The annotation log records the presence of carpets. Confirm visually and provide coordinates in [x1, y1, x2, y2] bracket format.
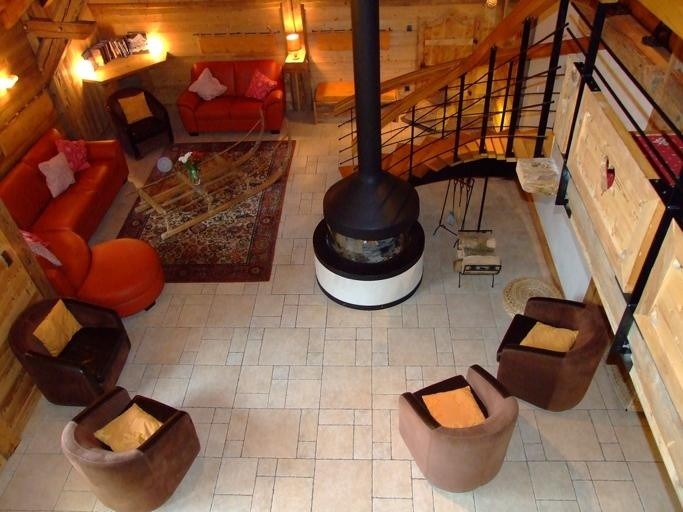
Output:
[503, 277, 565, 319]
[115, 141, 295, 284]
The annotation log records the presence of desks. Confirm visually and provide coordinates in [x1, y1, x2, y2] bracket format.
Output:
[81, 49, 172, 136]
[282, 61, 313, 119]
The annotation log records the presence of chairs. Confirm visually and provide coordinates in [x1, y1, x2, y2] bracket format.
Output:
[8, 297, 132, 407]
[61, 385, 199, 512]
[496, 296, 607, 412]
[452, 230, 500, 287]
[397, 364, 519, 493]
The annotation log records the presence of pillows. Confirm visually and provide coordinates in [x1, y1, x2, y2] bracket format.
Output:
[188, 68, 228, 101]
[243, 70, 278, 99]
[34, 300, 83, 357]
[421, 385, 485, 428]
[107, 87, 175, 161]
[18, 229, 63, 267]
[93, 402, 165, 452]
[520, 321, 579, 353]
[37, 151, 76, 198]
[54, 139, 91, 172]
[118, 92, 155, 125]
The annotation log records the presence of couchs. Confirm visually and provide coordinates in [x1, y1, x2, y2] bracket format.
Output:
[177, 58, 286, 136]
[0, 126, 165, 316]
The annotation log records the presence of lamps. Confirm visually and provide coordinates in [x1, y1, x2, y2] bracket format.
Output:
[285, 34, 302, 60]
